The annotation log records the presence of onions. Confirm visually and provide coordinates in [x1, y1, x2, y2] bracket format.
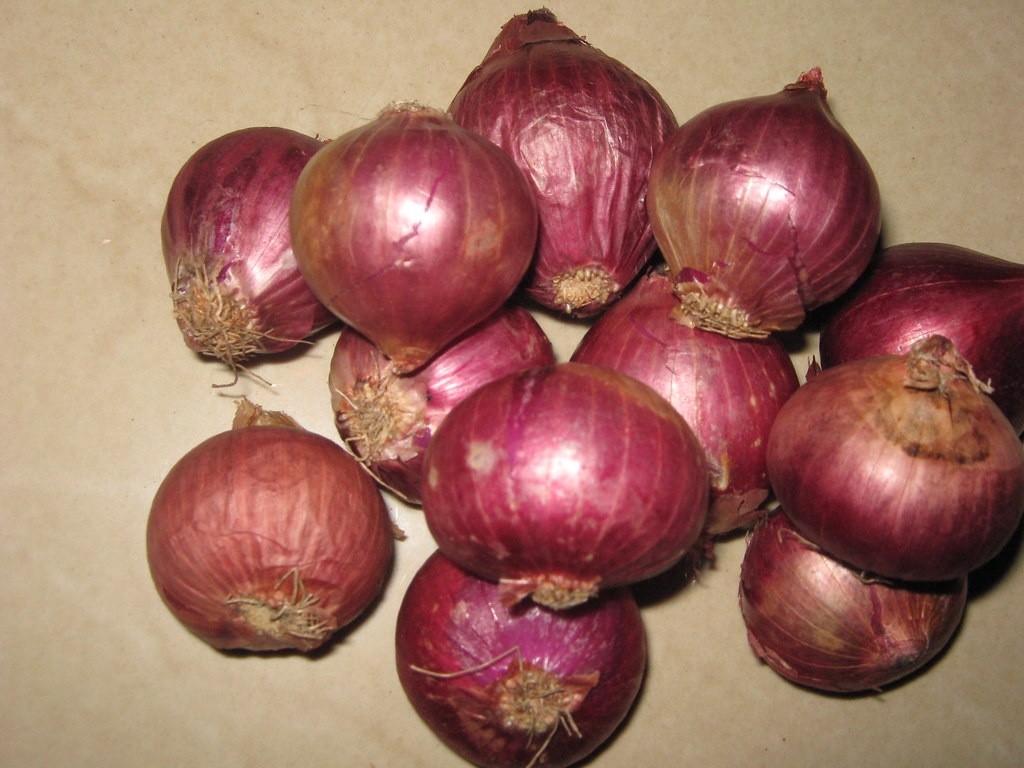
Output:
[145, 7, 1024, 768]
